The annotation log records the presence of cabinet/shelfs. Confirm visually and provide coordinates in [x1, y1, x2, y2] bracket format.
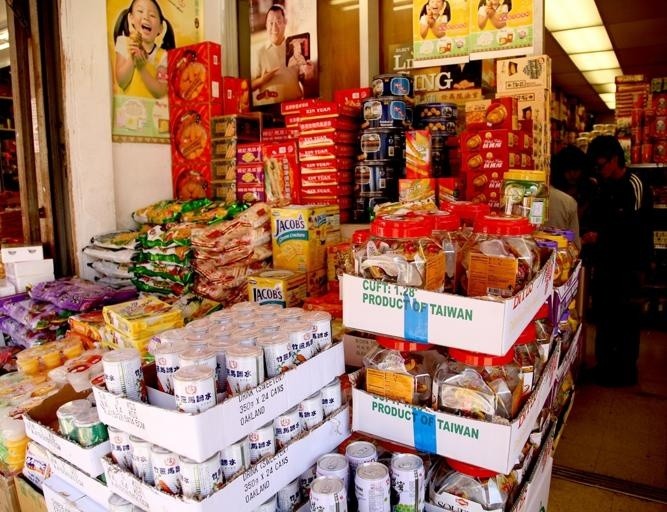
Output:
[625, 163, 666, 329]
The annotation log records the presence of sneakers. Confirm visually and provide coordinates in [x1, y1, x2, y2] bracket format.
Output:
[582, 362, 638, 388]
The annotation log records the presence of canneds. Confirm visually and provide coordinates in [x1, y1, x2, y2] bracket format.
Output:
[55, 301, 426, 512]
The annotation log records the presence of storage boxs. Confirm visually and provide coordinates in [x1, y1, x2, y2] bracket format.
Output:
[168, 43, 554, 220]
[23, 203, 585, 512]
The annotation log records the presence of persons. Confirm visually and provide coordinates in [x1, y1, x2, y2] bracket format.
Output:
[549, 116, 588, 198]
[576, 134, 655, 389]
[557, 142, 600, 327]
[419, 0, 452, 39]
[476, 1, 513, 30]
[255, 3, 308, 79]
[113, 1, 177, 101]
